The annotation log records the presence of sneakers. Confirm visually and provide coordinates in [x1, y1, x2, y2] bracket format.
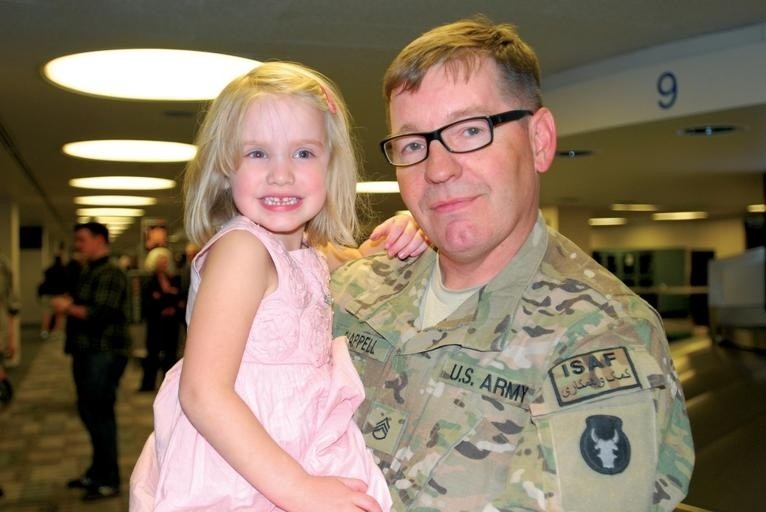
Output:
[66, 471, 120, 504]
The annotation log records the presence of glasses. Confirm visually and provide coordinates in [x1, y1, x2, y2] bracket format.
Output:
[379, 110, 534, 167]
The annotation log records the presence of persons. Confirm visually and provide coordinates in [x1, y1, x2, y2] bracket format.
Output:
[126, 58, 441, 512]
[1, 218, 203, 507]
[324, 19, 697, 512]
